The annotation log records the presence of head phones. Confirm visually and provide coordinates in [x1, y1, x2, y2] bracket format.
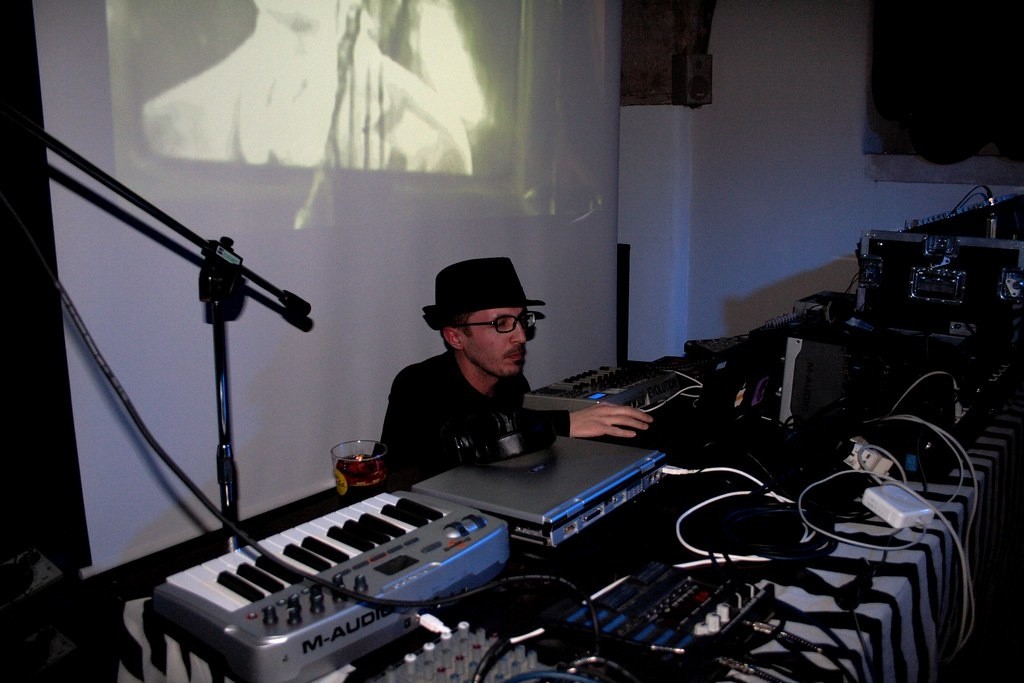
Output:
[439, 409, 558, 465]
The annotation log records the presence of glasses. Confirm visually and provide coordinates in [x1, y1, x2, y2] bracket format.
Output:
[452, 311, 536, 333]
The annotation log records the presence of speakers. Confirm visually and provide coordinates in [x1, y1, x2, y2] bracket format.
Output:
[671, 54, 712, 105]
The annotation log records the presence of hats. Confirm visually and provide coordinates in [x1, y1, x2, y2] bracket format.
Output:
[422, 257, 546, 330]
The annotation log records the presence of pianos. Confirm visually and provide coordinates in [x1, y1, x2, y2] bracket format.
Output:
[150, 490, 509, 683]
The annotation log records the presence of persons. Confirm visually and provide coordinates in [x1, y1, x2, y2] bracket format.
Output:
[380, 257, 652, 481]
[141, 0, 474, 176]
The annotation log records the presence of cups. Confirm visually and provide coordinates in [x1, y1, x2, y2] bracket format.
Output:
[331, 440, 389, 505]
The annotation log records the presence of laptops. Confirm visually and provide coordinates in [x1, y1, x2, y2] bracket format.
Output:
[411, 434, 667, 546]
